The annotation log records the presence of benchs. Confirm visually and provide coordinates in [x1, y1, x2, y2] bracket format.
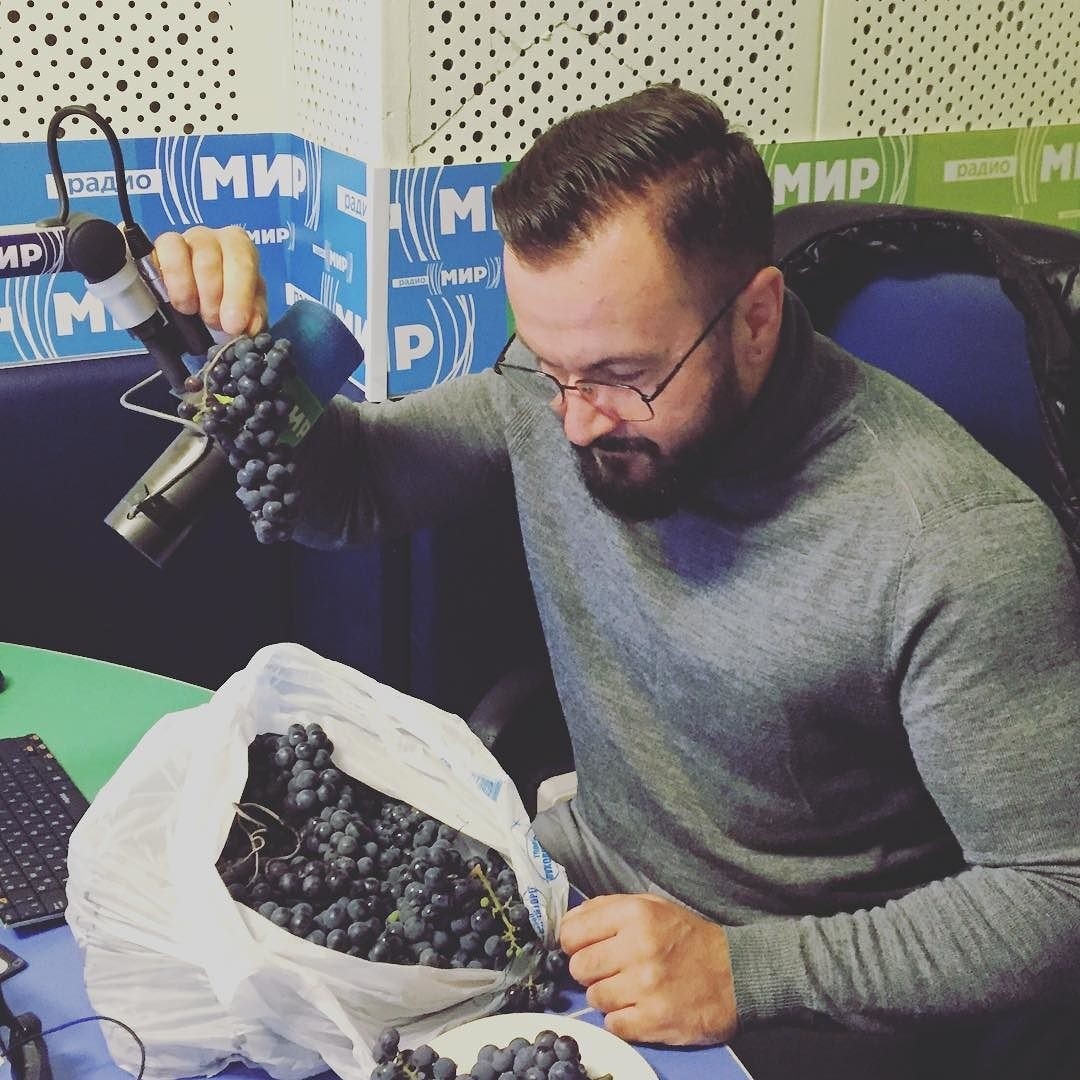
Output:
[1, 640, 757, 1080]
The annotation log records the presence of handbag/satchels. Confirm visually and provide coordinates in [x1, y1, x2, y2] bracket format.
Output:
[64, 644, 568, 1080]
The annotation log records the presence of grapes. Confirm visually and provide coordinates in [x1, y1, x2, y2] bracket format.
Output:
[216, 722, 567, 1015]
[178, 331, 308, 545]
[370, 1026, 613, 1080]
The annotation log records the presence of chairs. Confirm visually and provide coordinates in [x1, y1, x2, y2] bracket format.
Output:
[470, 200, 1080, 807]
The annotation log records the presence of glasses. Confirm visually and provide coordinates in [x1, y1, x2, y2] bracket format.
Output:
[492, 296, 733, 425]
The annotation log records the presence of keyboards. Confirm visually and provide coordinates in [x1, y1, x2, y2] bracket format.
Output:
[0, 733, 92, 925]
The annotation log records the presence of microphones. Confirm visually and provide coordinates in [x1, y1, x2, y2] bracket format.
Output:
[104, 300, 365, 568]
[125, 222, 213, 355]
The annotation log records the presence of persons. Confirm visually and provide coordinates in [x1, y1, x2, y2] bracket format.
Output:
[155, 83, 1080, 1080]
